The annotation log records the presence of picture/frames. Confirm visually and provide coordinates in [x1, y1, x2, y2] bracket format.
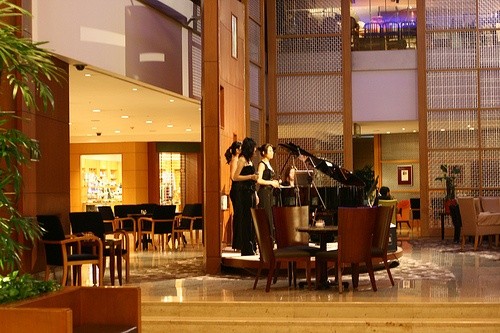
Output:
[396, 164, 413, 186]
[231, 12, 239, 60]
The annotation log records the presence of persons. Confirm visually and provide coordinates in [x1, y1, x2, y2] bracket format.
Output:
[373, 186, 393, 208]
[233, 138, 260, 256]
[280, 165, 298, 186]
[256, 143, 282, 206]
[225, 141, 242, 252]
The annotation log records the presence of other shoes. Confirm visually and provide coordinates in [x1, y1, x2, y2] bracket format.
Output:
[241, 250, 256, 255]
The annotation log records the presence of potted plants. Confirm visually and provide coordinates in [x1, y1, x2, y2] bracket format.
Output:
[434, 165, 462, 214]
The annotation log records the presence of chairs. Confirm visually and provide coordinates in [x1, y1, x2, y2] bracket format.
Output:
[250, 205, 394, 294]
[35, 203, 203, 287]
[457, 197, 500, 251]
[409, 198, 420, 232]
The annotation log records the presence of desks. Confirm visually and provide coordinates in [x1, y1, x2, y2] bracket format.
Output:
[295, 226, 349, 290]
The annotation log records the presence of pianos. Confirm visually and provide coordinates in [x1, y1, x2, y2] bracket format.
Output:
[272, 142, 367, 225]
